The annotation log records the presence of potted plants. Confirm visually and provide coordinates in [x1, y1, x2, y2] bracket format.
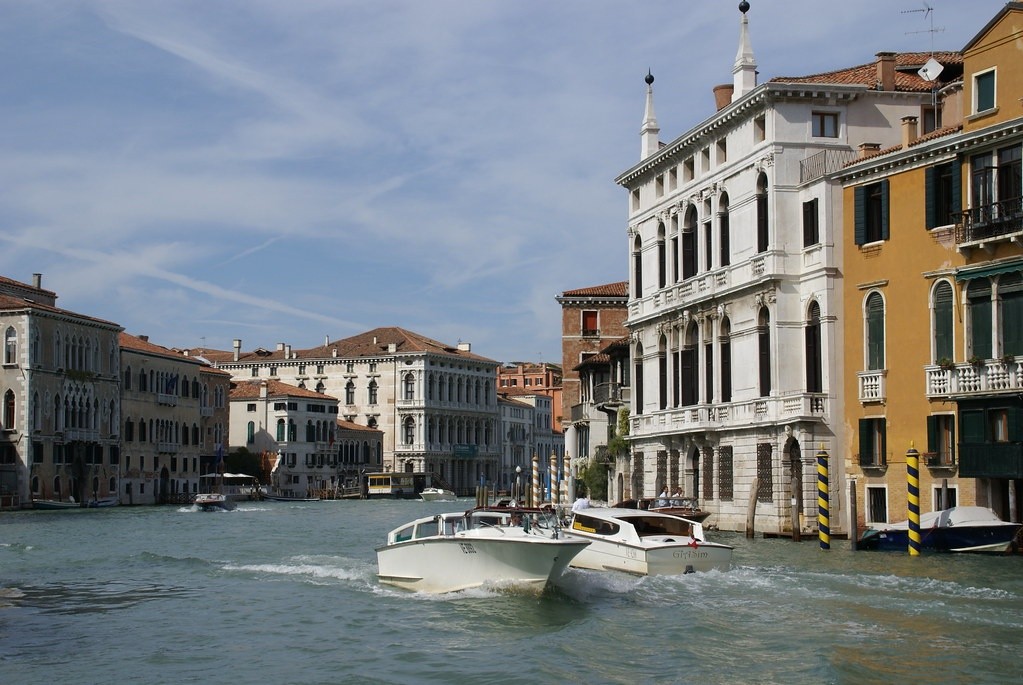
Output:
[935, 357, 954, 371]
[968, 355, 985, 367]
[1000, 354, 1015, 365]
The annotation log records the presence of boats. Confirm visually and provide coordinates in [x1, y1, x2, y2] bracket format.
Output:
[856, 505, 1023, 558]
[32, 497, 118, 511]
[419, 487, 459, 502]
[467, 496, 736, 591]
[373, 500, 592, 600]
[193, 493, 227, 511]
[363, 472, 416, 500]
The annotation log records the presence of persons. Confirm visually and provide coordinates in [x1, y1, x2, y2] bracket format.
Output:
[511, 516, 521, 527]
[659, 485, 670, 508]
[569, 492, 590, 521]
[670, 487, 684, 508]
